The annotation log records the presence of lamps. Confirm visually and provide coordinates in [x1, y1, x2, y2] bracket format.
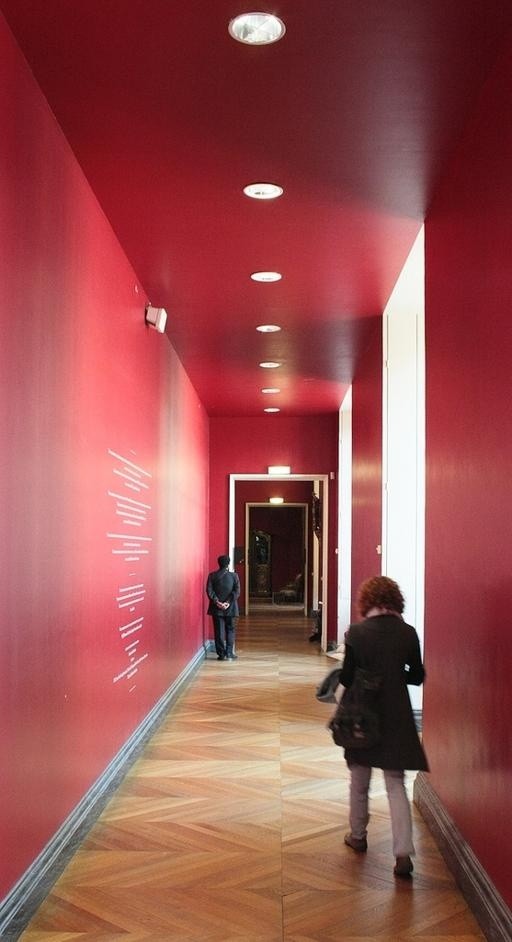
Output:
[144, 305, 168, 334]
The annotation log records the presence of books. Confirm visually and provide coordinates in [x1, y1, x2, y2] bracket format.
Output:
[325, 644, 346, 663]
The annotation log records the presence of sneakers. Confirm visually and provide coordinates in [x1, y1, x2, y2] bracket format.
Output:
[344, 833, 367, 851]
[393, 857, 413, 874]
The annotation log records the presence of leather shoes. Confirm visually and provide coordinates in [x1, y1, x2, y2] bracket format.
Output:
[217, 654, 238, 660]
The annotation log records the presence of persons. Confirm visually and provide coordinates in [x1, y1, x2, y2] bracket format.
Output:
[332, 574, 433, 877]
[204, 555, 242, 661]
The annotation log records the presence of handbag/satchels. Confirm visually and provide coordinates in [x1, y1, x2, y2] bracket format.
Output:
[327, 703, 366, 749]
